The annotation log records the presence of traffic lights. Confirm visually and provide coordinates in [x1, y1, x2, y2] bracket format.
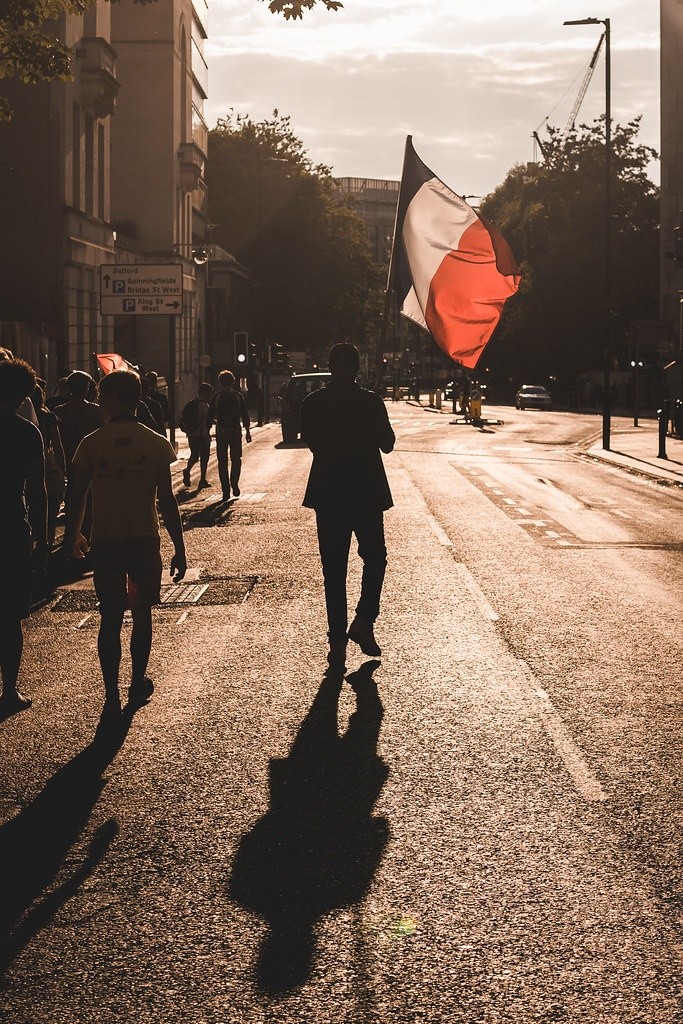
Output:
[234, 332, 248, 366]
[273, 343, 284, 367]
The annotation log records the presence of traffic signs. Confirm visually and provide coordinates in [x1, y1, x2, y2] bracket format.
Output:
[100, 264, 183, 315]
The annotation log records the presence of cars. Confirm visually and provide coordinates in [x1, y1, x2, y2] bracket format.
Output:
[515, 384, 552, 411]
[444, 387, 453, 400]
[280, 373, 337, 444]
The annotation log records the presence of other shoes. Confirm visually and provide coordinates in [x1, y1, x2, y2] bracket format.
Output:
[328, 641, 346, 663]
[101, 701, 121, 720]
[0, 690, 32, 711]
[349, 615, 381, 656]
[223, 491, 230, 500]
[128, 677, 154, 702]
[183, 469, 190, 487]
[199, 482, 211, 488]
[231, 480, 240, 496]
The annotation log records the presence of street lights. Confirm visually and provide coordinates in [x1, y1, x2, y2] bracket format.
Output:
[631, 360, 644, 427]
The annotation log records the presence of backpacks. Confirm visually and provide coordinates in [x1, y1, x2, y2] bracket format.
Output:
[217, 390, 240, 426]
[179, 397, 209, 433]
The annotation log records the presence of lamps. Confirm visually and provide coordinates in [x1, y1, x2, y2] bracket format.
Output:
[174, 242, 207, 264]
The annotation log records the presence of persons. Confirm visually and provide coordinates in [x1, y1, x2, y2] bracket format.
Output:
[455, 369, 466, 414]
[456, 371, 472, 415]
[0, 346, 253, 716]
[298, 343, 395, 667]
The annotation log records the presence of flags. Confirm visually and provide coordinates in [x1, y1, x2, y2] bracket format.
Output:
[95, 352, 140, 377]
[386, 135, 524, 368]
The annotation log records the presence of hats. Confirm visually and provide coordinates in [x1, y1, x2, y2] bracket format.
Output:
[64, 370, 92, 393]
[57, 377, 68, 389]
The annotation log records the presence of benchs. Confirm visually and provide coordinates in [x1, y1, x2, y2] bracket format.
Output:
[385, 386, 410, 399]
